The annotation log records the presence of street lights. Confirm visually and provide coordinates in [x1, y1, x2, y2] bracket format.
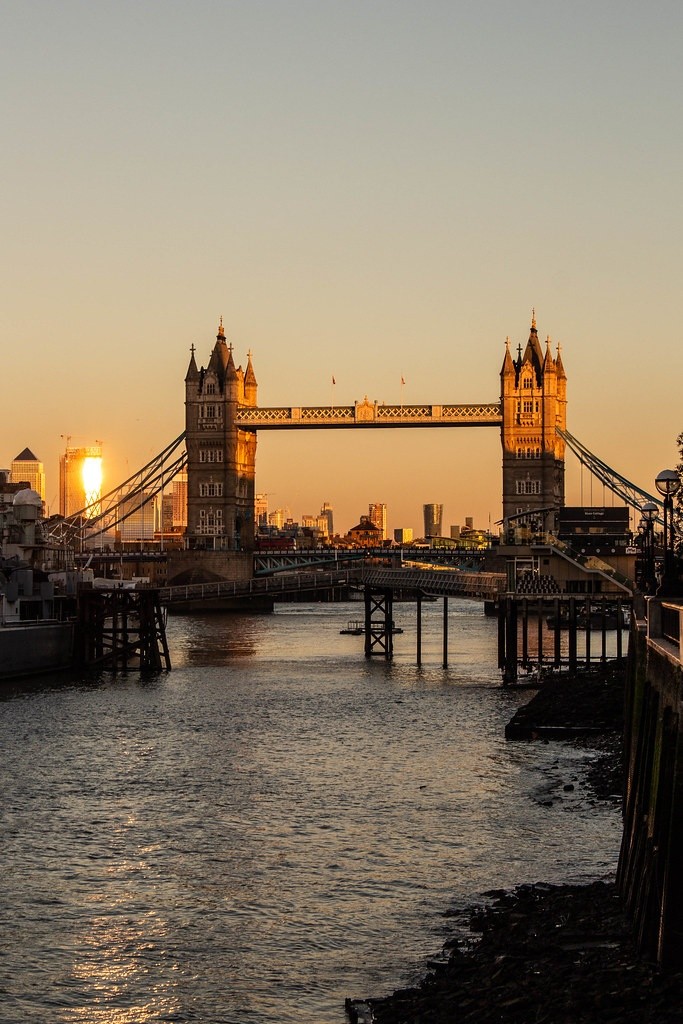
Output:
[639, 517, 649, 578]
[639, 502, 660, 595]
[654, 468, 677, 594]
[636, 525, 645, 581]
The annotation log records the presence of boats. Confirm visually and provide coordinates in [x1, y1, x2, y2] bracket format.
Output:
[546, 604, 638, 630]
[339, 620, 405, 636]
